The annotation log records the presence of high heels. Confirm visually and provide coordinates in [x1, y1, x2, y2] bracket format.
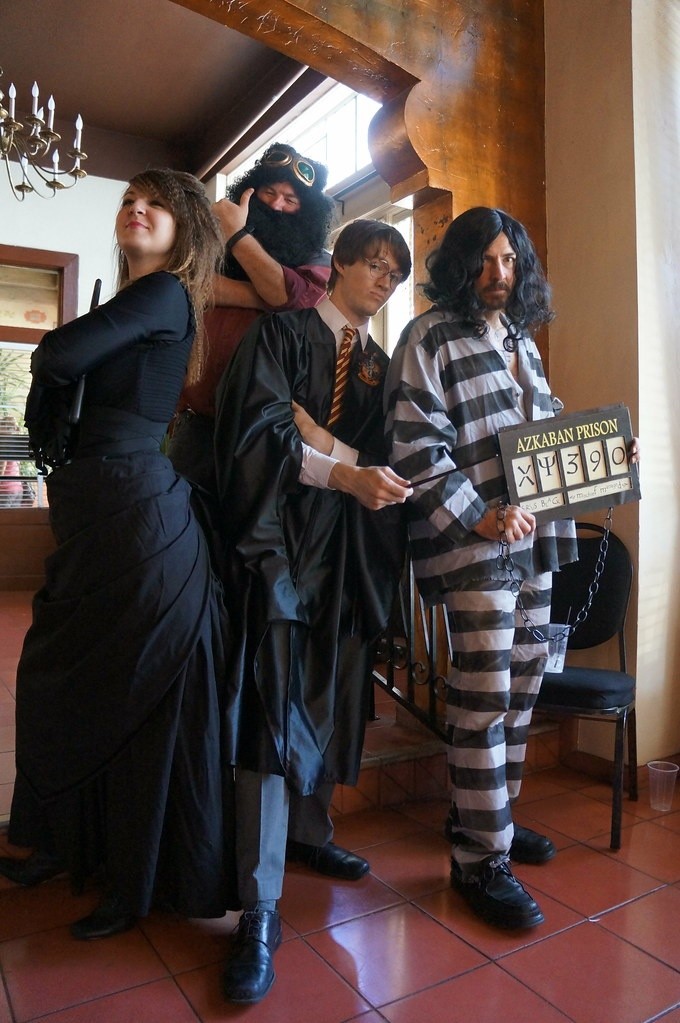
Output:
[64, 884, 164, 943]
[0, 849, 83, 897]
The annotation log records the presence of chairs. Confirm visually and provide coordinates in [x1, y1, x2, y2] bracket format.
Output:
[534, 522, 639, 850]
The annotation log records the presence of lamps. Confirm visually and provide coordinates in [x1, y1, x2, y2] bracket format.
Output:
[0, 66, 88, 202]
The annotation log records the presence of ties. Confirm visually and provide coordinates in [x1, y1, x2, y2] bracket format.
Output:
[323, 324, 358, 432]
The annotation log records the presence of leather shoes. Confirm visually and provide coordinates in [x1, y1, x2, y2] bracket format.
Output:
[218, 908, 283, 1006]
[286, 840, 370, 882]
[449, 855, 545, 932]
[444, 814, 557, 867]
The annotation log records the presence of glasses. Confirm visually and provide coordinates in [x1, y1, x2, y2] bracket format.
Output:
[360, 254, 406, 292]
[263, 150, 317, 187]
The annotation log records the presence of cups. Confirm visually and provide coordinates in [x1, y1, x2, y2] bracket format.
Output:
[647, 760, 679, 811]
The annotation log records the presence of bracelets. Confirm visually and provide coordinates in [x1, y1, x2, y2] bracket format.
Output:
[224, 223, 257, 250]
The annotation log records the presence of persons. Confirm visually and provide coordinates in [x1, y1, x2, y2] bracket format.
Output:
[167, 142, 331, 423]
[384, 204, 640, 936]
[195, 220, 415, 1005]
[1, 169, 229, 942]
